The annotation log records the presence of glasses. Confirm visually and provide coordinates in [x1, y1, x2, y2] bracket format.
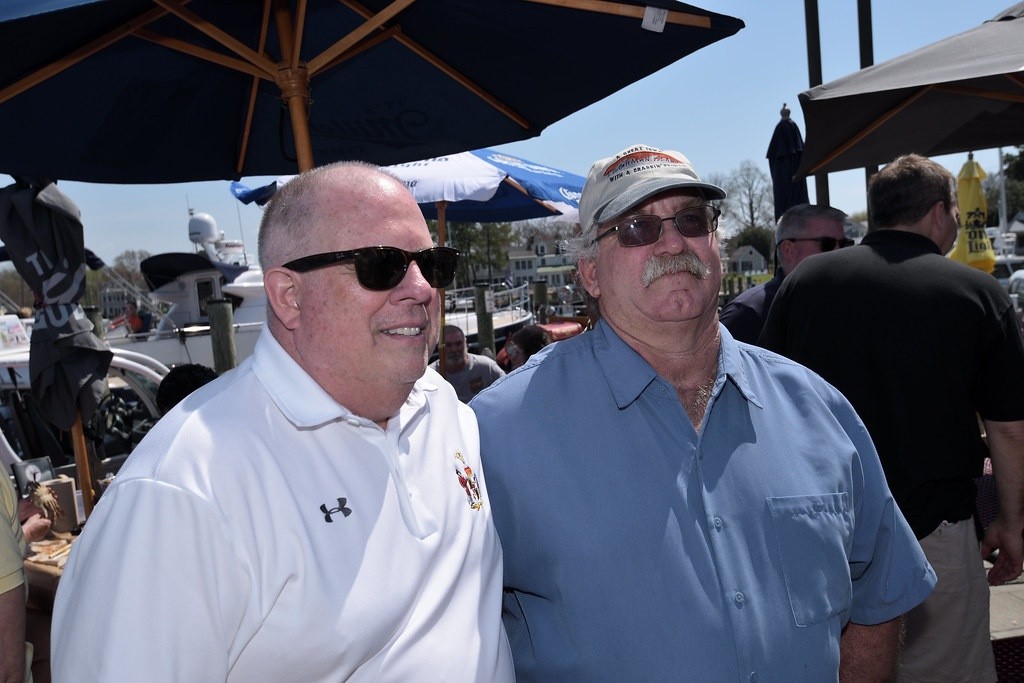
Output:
[775, 236, 858, 254]
[588, 204, 722, 248]
[280, 245, 461, 292]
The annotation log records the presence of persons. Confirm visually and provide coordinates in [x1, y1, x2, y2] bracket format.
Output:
[762, 153, 1024, 683]
[468, 144, 938, 683]
[720, 205, 854, 346]
[0, 462, 51, 683]
[156, 363, 218, 417]
[428, 325, 505, 404]
[52, 160, 518, 682]
[508, 327, 548, 370]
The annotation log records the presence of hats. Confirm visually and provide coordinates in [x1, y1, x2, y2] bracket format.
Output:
[577, 145, 726, 235]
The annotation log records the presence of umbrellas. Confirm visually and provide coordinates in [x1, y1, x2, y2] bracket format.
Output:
[793, 0, 1024, 180]
[765, 104, 811, 223]
[229, 150, 585, 380]
[948, 151, 996, 274]
[0, 0, 745, 185]
[0, 182, 114, 519]
